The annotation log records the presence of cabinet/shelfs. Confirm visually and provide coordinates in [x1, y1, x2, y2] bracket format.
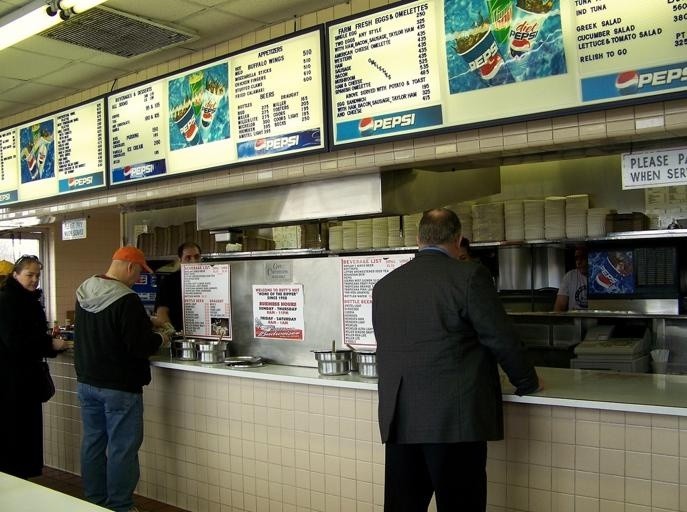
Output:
[470, 229, 687, 323]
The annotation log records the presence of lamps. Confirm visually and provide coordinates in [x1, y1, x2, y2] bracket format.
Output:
[0, 0, 110, 52]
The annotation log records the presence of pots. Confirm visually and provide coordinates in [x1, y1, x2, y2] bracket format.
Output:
[497, 245, 532, 295]
[532, 243, 566, 295]
[174, 340, 378, 378]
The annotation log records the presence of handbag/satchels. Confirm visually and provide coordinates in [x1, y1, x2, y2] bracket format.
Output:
[40, 362, 55, 402]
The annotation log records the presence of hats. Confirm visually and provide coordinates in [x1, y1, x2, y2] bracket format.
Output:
[111, 246, 153, 273]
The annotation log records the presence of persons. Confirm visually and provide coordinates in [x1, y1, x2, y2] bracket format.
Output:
[155, 243, 201, 329]
[372, 209, 544, 512]
[553, 247, 588, 311]
[73, 246, 170, 512]
[0, 260, 14, 289]
[455, 239, 472, 263]
[0, 255, 68, 481]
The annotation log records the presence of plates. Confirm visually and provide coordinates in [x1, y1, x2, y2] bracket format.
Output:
[328, 194, 616, 250]
[636, 247, 676, 286]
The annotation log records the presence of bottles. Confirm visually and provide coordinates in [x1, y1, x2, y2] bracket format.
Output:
[65, 319, 71, 331]
[52, 321, 60, 338]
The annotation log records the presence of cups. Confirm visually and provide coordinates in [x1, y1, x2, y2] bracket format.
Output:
[457, 0, 549, 87]
[174, 77, 224, 147]
[593, 257, 627, 292]
[25, 128, 52, 180]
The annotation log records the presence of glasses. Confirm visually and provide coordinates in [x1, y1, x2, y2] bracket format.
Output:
[17, 254, 38, 264]
